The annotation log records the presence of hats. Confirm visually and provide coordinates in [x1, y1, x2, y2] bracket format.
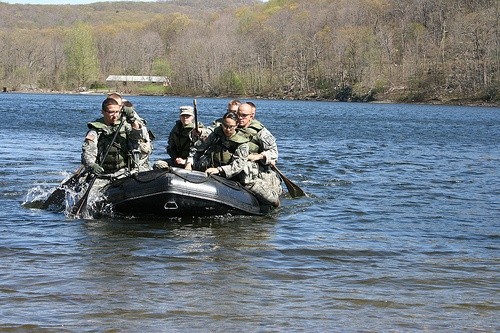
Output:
[179, 106, 194, 116]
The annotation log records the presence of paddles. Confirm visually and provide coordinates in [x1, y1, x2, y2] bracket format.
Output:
[70, 113, 129, 214]
[41, 167, 88, 209]
[192, 98, 199, 140]
[272, 163, 309, 199]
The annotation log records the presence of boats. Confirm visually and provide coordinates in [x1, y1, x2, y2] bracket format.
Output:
[102, 167, 268, 219]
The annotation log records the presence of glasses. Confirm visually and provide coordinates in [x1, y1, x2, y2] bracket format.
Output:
[223, 122, 237, 128]
[237, 111, 252, 117]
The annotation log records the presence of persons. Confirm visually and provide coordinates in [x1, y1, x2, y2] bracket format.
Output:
[81, 92, 281, 208]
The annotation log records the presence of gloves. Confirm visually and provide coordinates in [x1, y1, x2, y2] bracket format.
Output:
[122, 106, 137, 124]
[91, 164, 104, 176]
